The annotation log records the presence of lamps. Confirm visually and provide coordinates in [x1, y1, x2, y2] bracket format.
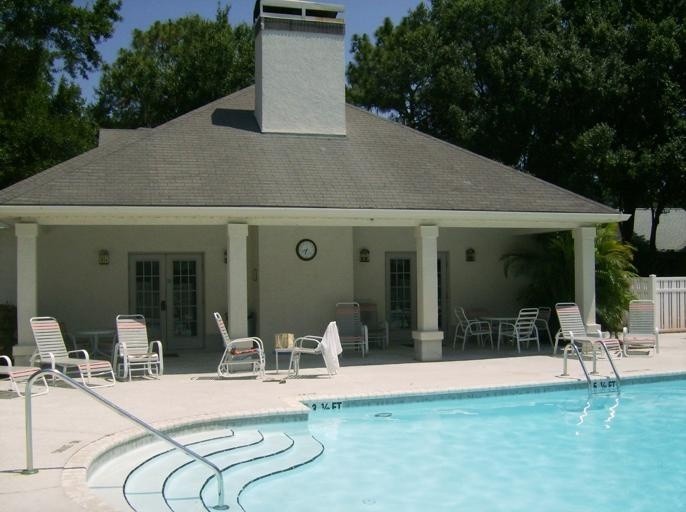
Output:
[360, 248, 370, 262]
[465, 248, 475, 262]
[223, 250, 227, 263]
[97, 249, 109, 265]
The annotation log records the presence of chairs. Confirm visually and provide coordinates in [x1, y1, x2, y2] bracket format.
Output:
[553, 301, 623, 361]
[1, 353, 50, 398]
[274, 333, 297, 373]
[213, 311, 266, 379]
[498, 308, 542, 353]
[334, 302, 370, 359]
[288, 320, 344, 379]
[29, 316, 116, 389]
[355, 301, 389, 354]
[112, 314, 164, 382]
[453, 304, 495, 352]
[623, 300, 659, 359]
[537, 306, 554, 347]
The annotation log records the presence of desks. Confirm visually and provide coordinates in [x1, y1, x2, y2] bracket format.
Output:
[479, 315, 532, 350]
[72, 328, 115, 361]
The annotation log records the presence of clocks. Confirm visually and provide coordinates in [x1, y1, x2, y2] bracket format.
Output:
[295, 239, 317, 261]
